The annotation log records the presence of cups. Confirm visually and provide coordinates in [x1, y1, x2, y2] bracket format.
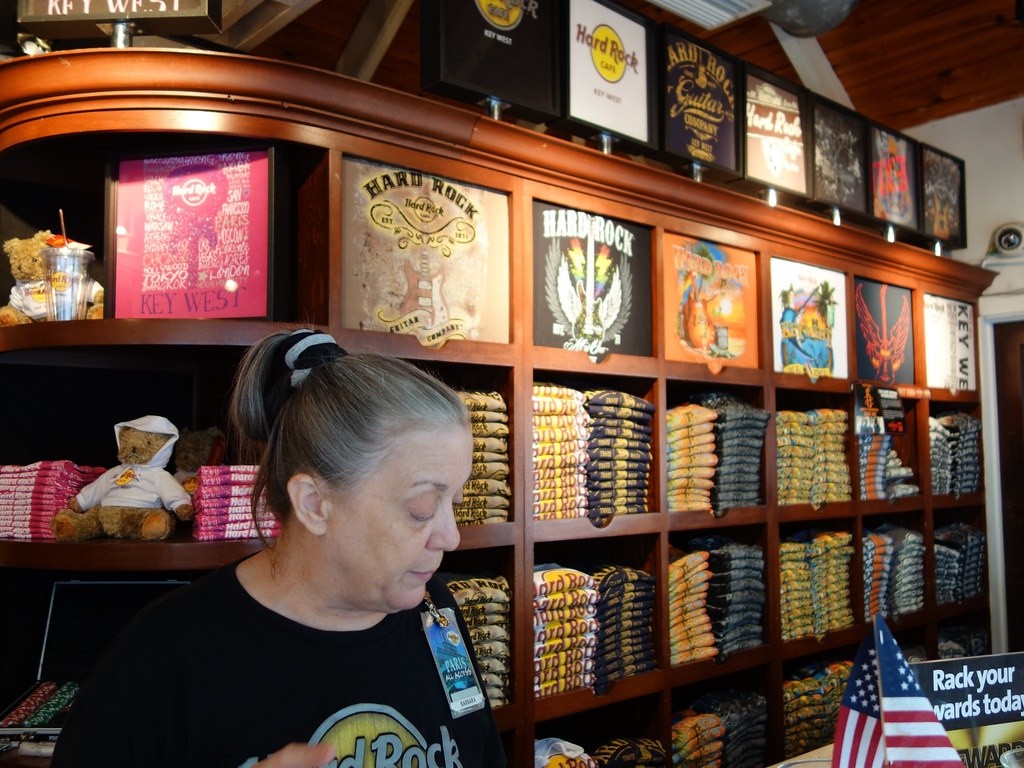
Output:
[40, 208, 95, 321]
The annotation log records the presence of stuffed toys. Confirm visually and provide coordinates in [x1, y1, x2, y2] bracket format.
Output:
[50, 414, 195, 541]
[170, 425, 227, 526]
[0, 228, 106, 325]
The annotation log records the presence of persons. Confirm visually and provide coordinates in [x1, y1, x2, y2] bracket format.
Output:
[52, 329, 510, 766]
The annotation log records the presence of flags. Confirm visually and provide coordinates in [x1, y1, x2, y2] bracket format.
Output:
[828, 611, 969, 768]
